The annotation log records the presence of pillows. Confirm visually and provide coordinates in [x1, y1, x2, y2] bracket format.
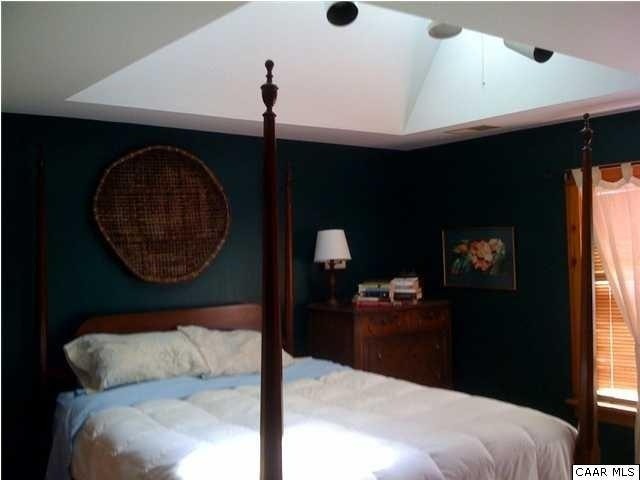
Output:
[62, 330, 210, 394]
[177, 324, 291, 379]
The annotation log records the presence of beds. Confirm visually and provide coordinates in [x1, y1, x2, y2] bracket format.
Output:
[32, 60, 599, 480]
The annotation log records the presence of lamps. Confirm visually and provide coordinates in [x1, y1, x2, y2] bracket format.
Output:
[327, 2, 359, 27]
[504, 39, 552, 63]
[429, 20, 461, 42]
[315, 228, 352, 307]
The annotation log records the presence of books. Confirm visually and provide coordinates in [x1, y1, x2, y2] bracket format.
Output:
[353, 276, 422, 308]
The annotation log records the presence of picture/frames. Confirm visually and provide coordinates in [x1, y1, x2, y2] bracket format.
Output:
[441, 226, 518, 292]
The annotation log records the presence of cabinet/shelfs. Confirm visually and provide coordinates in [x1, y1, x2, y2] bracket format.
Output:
[304, 300, 454, 389]
